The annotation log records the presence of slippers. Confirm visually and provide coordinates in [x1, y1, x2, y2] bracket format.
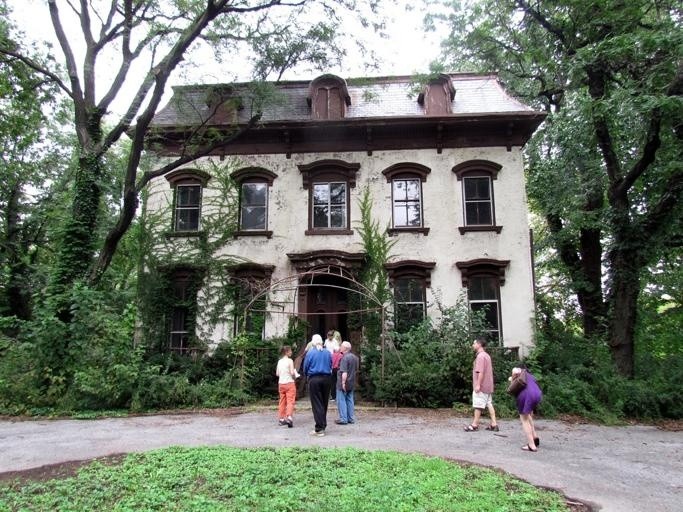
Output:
[522, 444, 537, 451]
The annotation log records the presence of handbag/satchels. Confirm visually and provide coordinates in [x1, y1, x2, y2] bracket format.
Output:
[331, 353, 343, 368]
[507, 371, 527, 397]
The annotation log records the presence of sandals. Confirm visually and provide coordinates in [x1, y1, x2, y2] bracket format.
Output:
[464, 425, 479, 431]
[486, 424, 499, 430]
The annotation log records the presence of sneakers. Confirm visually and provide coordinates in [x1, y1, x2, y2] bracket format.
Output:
[279, 418, 287, 425]
[309, 430, 326, 437]
[285, 419, 293, 428]
[335, 418, 354, 425]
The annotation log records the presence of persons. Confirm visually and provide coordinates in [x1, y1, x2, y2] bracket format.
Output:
[334, 340, 358, 425]
[463, 339, 499, 431]
[303, 334, 334, 437]
[506, 363, 543, 451]
[276, 345, 299, 428]
[327, 331, 343, 403]
[324, 328, 339, 360]
